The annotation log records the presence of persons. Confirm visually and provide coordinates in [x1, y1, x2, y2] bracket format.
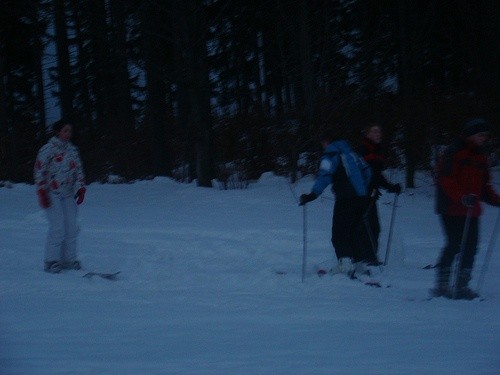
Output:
[298, 121, 401, 277]
[33, 119, 87, 273]
[432, 119, 500, 300]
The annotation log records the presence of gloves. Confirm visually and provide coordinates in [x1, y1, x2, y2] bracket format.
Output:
[298, 192, 310, 205]
[388, 183, 401, 195]
[38, 189, 51, 207]
[74, 187, 86, 204]
[463, 192, 479, 208]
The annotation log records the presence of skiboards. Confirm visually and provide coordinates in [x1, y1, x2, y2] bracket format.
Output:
[40, 258, 121, 280]
[278, 259, 489, 305]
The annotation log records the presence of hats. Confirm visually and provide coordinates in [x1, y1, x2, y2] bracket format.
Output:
[463, 115, 487, 137]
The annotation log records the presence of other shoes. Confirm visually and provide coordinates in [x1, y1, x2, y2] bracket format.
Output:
[60, 259, 81, 269]
[435, 289, 478, 300]
[356, 262, 379, 277]
[330, 258, 353, 274]
[44, 262, 61, 272]
